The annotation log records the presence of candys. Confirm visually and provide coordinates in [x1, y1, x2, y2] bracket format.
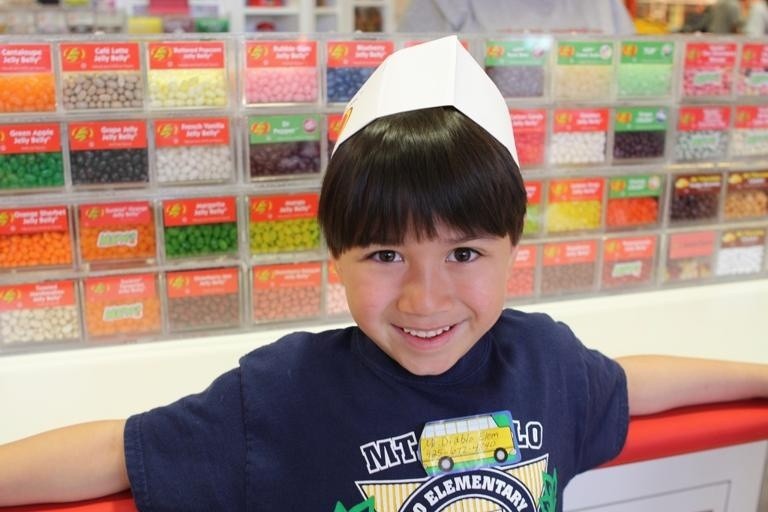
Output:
[0, 63, 768, 346]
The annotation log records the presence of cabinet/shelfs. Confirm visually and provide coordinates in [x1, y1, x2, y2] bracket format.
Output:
[237, 1, 393, 32]
[563, 438, 767, 511]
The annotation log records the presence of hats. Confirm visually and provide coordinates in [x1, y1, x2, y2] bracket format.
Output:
[330, 34, 518, 168]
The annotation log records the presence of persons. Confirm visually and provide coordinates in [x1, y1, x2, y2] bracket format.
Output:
[2, 106, 768, 512]
[680, 1, 768, 36]
[393, 0, 640, 33]
